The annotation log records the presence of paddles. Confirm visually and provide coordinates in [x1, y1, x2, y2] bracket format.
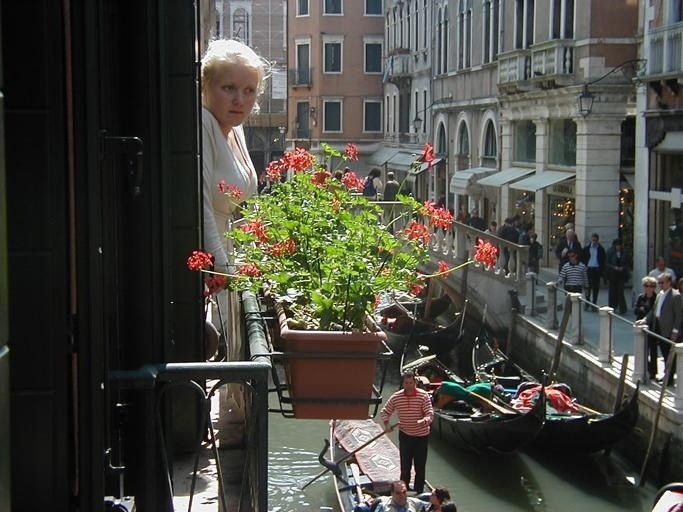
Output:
[467, 391, 517, 416]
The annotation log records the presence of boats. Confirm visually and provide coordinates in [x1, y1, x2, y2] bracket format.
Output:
[652, 479, 681, 512]
[398, 340, 641, 454]
[320, 409, 437, 511]
[369, 270, 470, 353]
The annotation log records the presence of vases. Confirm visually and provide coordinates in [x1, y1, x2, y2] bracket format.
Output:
[272, 298, 390, 424]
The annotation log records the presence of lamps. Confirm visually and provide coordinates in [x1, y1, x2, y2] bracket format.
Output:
[413, 97, 439, 130]
[575, 55, 649, 118]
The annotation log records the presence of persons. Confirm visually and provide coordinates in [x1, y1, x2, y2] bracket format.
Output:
[374, 480, 430, 511]
[380, 372, 434, 494]
[200, 39, 263, 281]
[424, 485, 450, 511]
[440, 501, 457, 512]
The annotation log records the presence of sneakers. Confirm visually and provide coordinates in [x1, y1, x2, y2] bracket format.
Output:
[647, 372, 675, 388]
[584, 301, 628, 314]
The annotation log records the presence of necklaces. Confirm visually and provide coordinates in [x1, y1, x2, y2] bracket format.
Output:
[224, 134, 234, 150]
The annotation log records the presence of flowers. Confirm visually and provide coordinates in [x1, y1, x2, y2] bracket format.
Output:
[185, 141, 501, 327]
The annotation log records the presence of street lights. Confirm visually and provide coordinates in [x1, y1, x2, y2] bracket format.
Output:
[277, 124, 285, 154]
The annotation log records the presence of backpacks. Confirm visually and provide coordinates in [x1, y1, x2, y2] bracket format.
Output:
[363, 175, 378, 195]
